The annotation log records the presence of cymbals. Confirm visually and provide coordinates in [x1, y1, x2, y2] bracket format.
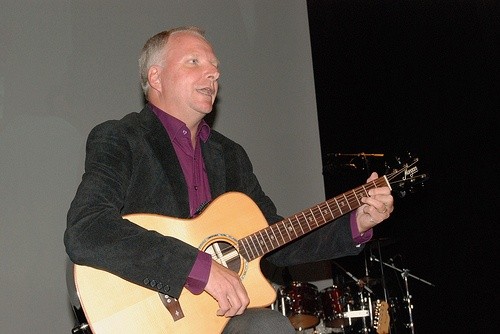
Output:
[363, 237, 389, 243]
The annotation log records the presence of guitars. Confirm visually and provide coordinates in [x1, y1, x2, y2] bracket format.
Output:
[372, 298, 392, 334]
[73, 152, 432, 334]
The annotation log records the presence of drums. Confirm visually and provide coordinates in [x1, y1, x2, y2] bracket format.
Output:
[280, 278, 322, 331]
[320, 283, 356, 330]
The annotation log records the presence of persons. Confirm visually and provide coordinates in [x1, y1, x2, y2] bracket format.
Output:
[63, 27, 396, 334]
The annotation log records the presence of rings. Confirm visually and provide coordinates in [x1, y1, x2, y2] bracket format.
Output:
[379, 207, 388, 215]
